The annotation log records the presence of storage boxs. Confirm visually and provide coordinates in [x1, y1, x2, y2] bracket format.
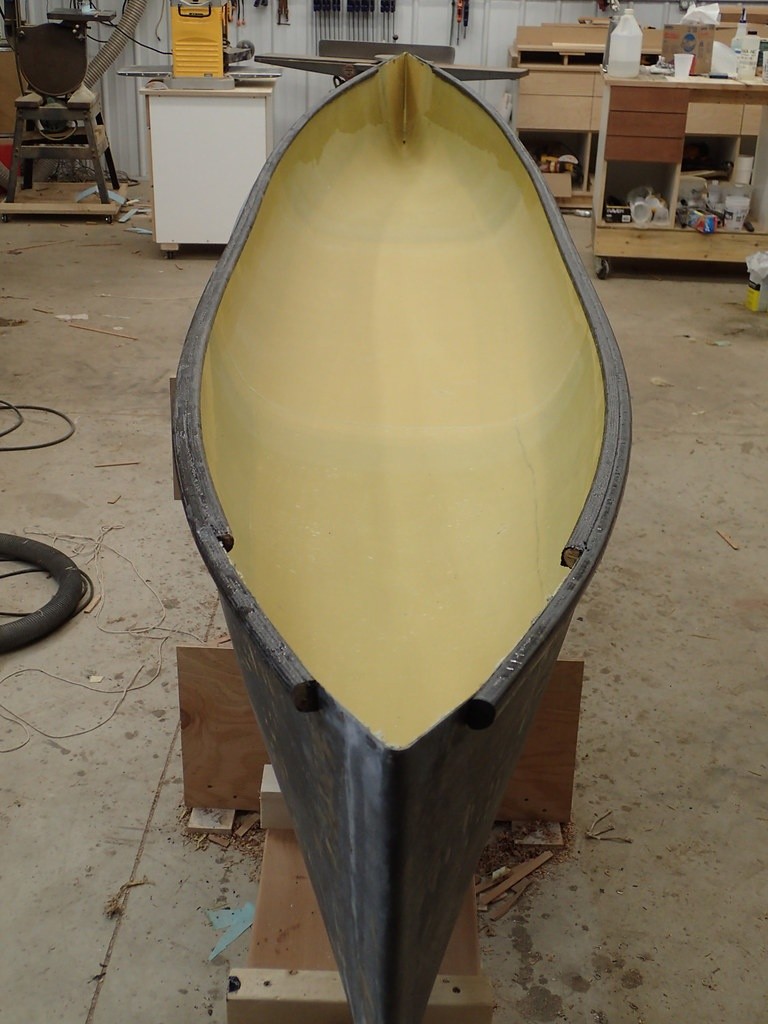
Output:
[662, 23, 716, 74]
[539, 155, 573, 198]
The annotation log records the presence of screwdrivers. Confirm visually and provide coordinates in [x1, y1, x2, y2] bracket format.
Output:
[456, 0, 463, 46]
[463, 0, 469, 39]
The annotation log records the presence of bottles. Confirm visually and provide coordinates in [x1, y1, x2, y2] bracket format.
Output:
[708, 180, 720, 207]
[731, 8, 747, 74]
[738, 30, 760, 81]
[608, 9, 643, 78]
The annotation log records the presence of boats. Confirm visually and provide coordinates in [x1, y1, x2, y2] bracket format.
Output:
[169, 49, 635, 1023]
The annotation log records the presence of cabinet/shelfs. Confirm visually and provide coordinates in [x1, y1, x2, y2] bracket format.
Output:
[507, 24, 768, 208]
[138, 78, 276, 260]
[589, 61, 768, 280]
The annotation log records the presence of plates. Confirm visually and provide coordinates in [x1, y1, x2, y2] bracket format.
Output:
[374, 54, 395, 61]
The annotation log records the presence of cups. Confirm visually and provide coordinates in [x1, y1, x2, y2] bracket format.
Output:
[674, 54, 693, 79]
[631, 202, 650, 223]
[724, 196, 750, 230]
[653, 208, 669, 225]
[763, 51, 768, 83]
